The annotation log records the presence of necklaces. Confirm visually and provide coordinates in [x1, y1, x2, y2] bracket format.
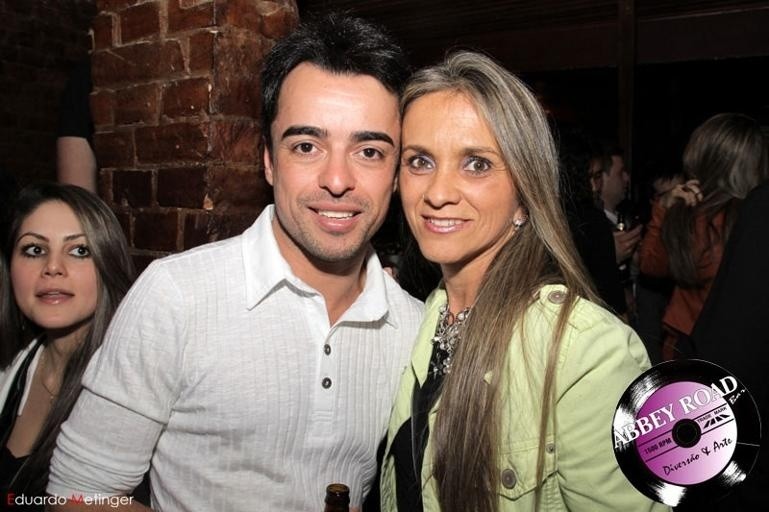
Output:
[432, 302, 473, 379]
[39, 352, 61, 406]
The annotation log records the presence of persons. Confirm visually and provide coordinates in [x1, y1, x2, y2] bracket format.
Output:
[568, 141, 644, 310]
[44, 9, 427, 512]
[0, 185, 136, 511]
[399, 44, 675, 512]
[634, 114, 766, 375]
[604, 152, 642, 321]
[5, 0, 97, 191]
